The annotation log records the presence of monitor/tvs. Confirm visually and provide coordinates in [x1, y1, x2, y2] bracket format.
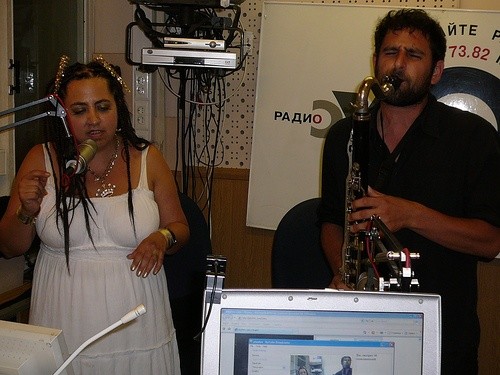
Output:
[0, 320, 74, 375]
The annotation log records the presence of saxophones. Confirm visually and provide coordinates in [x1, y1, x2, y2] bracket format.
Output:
[336, 72, 405, 295]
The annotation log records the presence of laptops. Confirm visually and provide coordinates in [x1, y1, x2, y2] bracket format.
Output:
[199, 288, 442, 375]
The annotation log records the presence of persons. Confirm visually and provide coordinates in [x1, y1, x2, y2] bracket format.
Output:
[298, 368, 308, 375]
[317, 8, 499, 375]
[334, 355, 353, 375]
[0, 58, 191, 375]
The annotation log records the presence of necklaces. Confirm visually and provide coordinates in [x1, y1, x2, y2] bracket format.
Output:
[71, 132, 120, 198]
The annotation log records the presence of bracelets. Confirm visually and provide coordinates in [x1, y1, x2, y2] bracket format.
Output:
[157, 226, 177, 250]
[16, 204, 40, 225]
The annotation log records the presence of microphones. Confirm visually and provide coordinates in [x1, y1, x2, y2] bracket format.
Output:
[53, 305, 146, 375]
[64, 139, 98, 178]
